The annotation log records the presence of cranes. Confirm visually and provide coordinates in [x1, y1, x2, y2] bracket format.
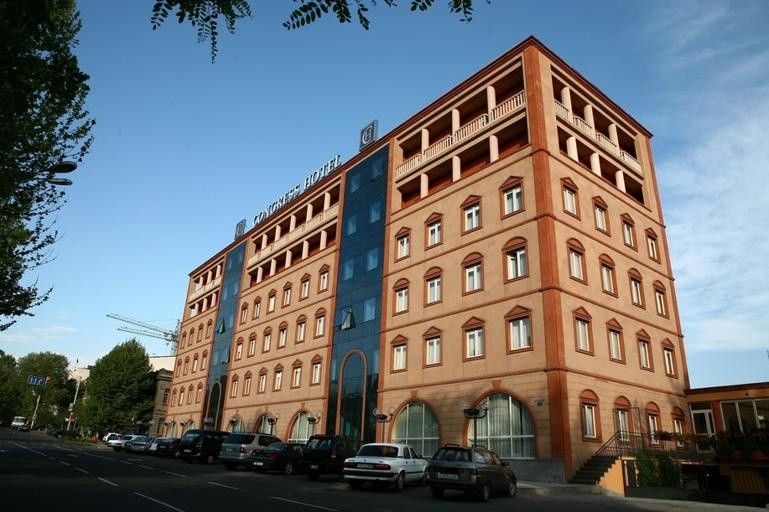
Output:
[104, 309, 182, 357]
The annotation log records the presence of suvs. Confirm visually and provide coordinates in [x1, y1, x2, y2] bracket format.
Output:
[217, 431, 285, 470]
[302, 433, 367, 481]
[422, 442, 519, 500]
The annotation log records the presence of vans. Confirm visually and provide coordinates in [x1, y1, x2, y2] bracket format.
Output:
[175, 429, 231, 464]
[10, 415, 28, 429]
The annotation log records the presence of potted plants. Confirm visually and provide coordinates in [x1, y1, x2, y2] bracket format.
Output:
[715, 431, 769, 463]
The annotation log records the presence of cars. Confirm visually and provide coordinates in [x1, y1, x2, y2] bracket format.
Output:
[101, 431, 181, 457]
[249, 442, 315, 476]
[342, 441, 429, 491]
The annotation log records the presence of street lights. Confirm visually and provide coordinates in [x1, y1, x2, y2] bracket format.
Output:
[456, 399, 494, 450]
[63, 368, 81, 432]
[202, 416, 215, 431]
[265, 412, 282, 435]
[135, 419, 191, 439]
[372, 406, 396, 443]
[304, 410, 324, 437]
[226, 415, 241, 433]
